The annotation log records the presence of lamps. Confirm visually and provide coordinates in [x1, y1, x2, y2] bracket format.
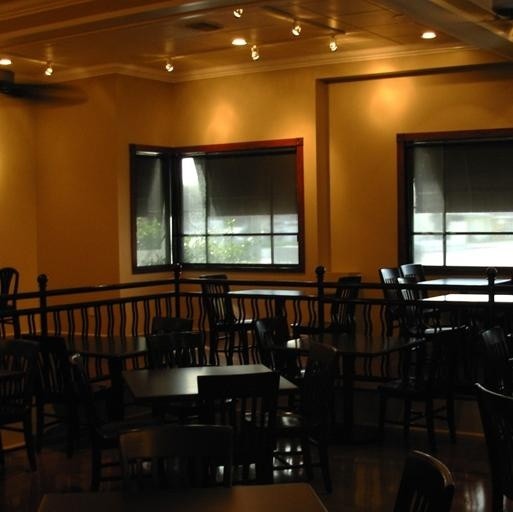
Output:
[233, 6, 337, 61]
